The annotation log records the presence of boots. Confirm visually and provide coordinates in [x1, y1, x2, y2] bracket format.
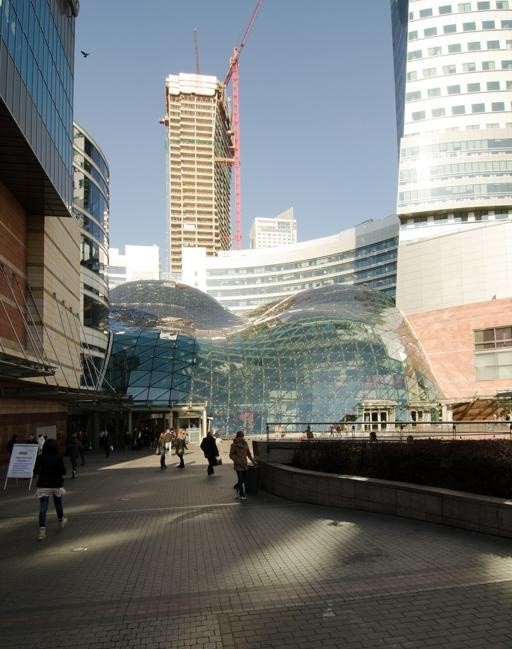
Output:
[38, 517, 68, 540]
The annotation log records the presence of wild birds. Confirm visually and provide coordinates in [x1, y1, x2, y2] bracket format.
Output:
[80, 50, 90, 57]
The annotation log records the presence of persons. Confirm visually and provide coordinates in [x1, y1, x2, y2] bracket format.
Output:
[229, 431, 258, 500]
[369, 431, 377, 443]
[32, 439, 67, 541]
[7, 425, 189, 479]
[215, 432, 223, 465]
[302, 426, 313, 438]
[200, 432, 218, 475]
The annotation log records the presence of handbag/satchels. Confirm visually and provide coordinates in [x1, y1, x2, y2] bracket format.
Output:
[212, 458, 223, 466]
[176, 448, 184, 457]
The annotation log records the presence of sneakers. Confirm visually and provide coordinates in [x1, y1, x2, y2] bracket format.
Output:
[234, 482, 247, 500]
[160, 464, 185, 470]
[71, 470, 78, 480]
[208, 469, 215, 475]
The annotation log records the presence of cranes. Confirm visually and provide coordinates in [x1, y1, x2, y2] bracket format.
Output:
[191, 0, 266, 249]
